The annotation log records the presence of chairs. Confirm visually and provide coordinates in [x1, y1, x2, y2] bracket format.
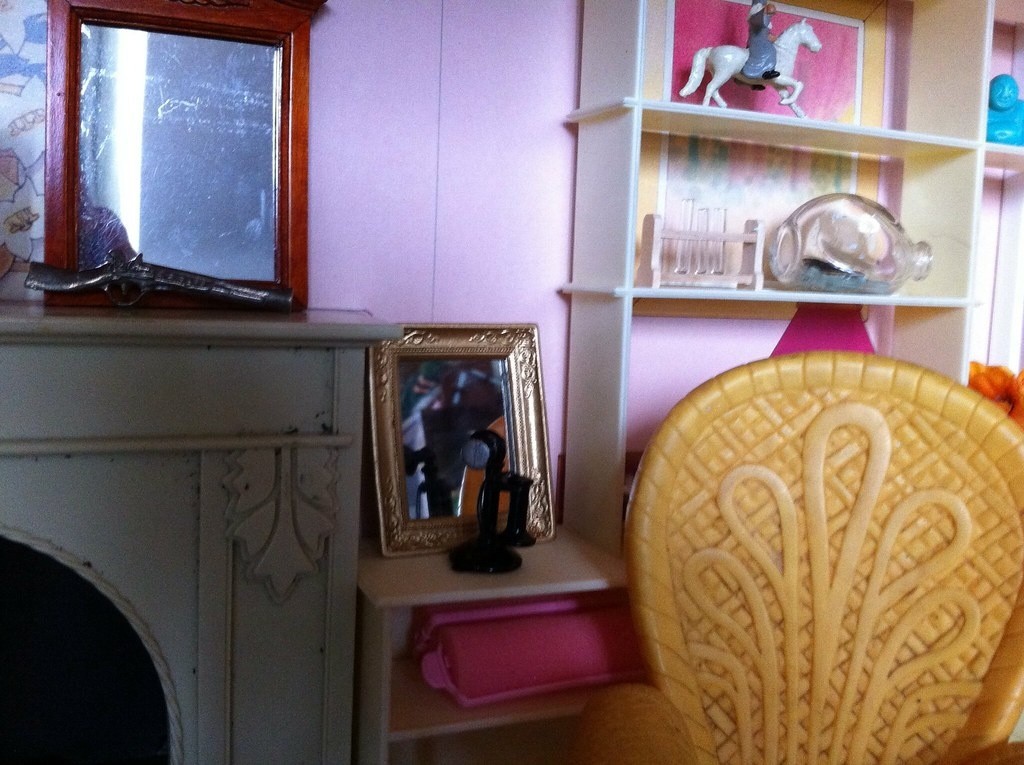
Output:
[577, 350, 1023, 764]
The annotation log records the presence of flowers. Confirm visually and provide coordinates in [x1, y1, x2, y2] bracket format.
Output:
[969, 361, 1023, 428]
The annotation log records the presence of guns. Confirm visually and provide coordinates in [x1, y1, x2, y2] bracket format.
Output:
[22, 249, 296, 318]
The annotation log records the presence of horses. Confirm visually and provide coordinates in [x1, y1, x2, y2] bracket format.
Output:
[678, 18, 823, 118]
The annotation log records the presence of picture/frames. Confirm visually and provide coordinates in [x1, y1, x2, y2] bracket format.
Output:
[657, 0, 865, 288]
[368, 322, 556, 558]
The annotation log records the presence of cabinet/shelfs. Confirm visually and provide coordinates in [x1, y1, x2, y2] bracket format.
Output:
[357, 526, 630, 765]
[562, 0, 1024, 558]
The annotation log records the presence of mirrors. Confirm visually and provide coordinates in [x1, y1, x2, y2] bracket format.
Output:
[44, 0, 324, 315]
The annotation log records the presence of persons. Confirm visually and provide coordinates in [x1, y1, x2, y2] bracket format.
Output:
[739, 0, 780, 91]
[986, 74, 1024, 147]
